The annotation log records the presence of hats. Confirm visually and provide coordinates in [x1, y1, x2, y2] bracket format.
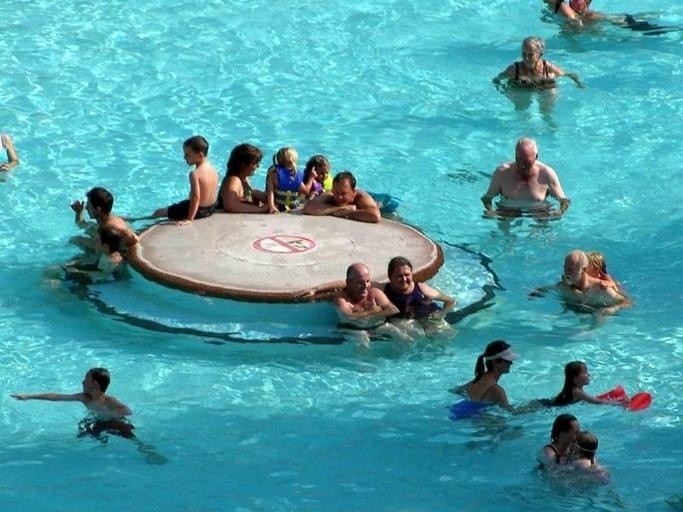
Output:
[486, 345, 521, 361]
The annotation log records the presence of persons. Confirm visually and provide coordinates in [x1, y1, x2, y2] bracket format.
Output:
[556, 250, 619, 297]
[488, 37, 582, 128]
[332, 265, 413, 352]
[9, 367, 168, 465]
[0, 135, 19, 173]
[460, 341, 521, 408]
[479, 140, 571, 237]
[71, 187, 138, 261]
[290, 256, 459, 362]
[217, 143, 381, 223]
[545, 414, 598, 468]
[553, 361, 625, 407]
[153, 135, 218, 226]
[566, 1, 624, 35]
[539, 0, 583, 31]
